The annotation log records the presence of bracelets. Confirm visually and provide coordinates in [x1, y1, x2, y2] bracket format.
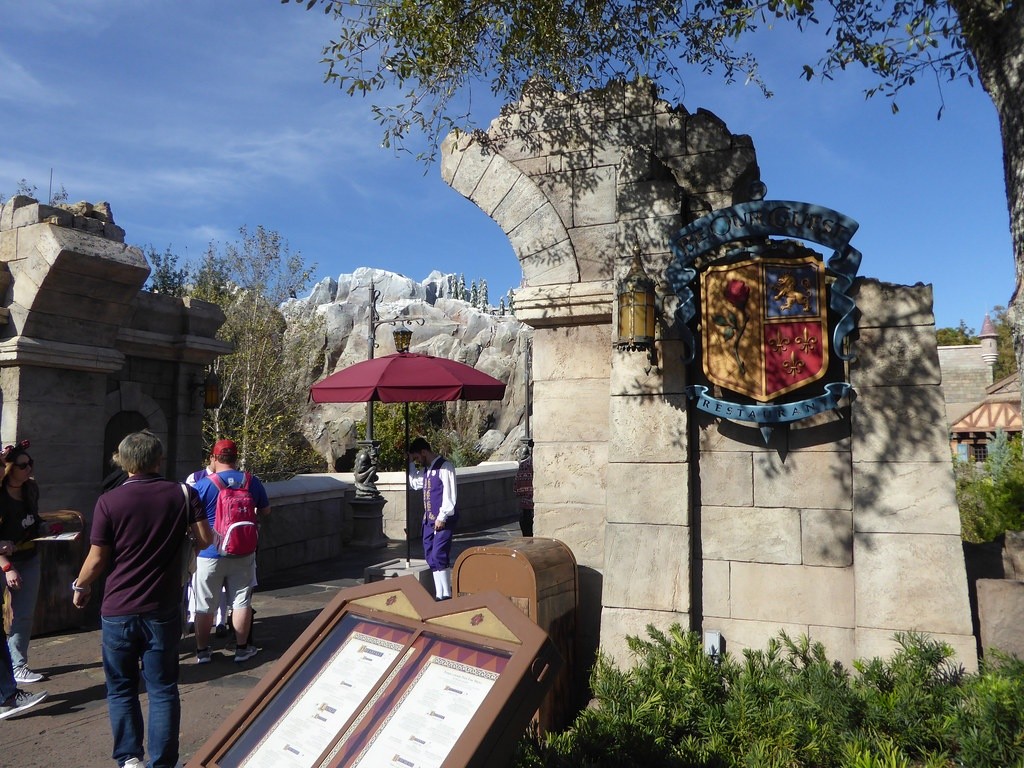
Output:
[2, 562, 14, 572]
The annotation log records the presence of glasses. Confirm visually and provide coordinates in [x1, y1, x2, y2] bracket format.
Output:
[14, 460, 34, 470]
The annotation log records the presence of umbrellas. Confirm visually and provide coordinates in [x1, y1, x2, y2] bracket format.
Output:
[307, 352, 508, 568]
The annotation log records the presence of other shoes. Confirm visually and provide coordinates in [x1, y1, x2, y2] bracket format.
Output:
[121, 758, 147, 768]
[216, 624, 226, 637]
[185, 622, 195, 633]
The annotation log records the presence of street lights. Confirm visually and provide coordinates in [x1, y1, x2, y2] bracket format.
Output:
[392, 321, 414, 569]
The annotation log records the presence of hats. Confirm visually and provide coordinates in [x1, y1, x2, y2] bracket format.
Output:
[210, 440, 238, 456]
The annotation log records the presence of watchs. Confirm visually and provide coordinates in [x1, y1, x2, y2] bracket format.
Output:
[73, 578, 90, 591]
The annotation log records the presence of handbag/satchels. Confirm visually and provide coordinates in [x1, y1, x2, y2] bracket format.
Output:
[179, 482, 198, 586]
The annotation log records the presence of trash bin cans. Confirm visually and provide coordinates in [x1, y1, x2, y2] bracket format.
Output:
[451, 537, 580, 739]
[3, 510, 89, 642]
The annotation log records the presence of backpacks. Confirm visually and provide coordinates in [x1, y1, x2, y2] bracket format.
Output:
[206, 471, 260, 558]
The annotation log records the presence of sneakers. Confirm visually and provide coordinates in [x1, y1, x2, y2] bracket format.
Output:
[234, 644, 258, 662]
[197, 646, 213, 663]
[13, 664, 44, 682]
[0, 689, 48, 718]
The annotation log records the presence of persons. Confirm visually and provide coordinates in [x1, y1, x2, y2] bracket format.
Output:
[0, 440, 61, 720]
[185, 439, 271, 663]
[513, 456, 533, 537]
[72, 428, 214, 768]
[407, 438, 458, 601]
